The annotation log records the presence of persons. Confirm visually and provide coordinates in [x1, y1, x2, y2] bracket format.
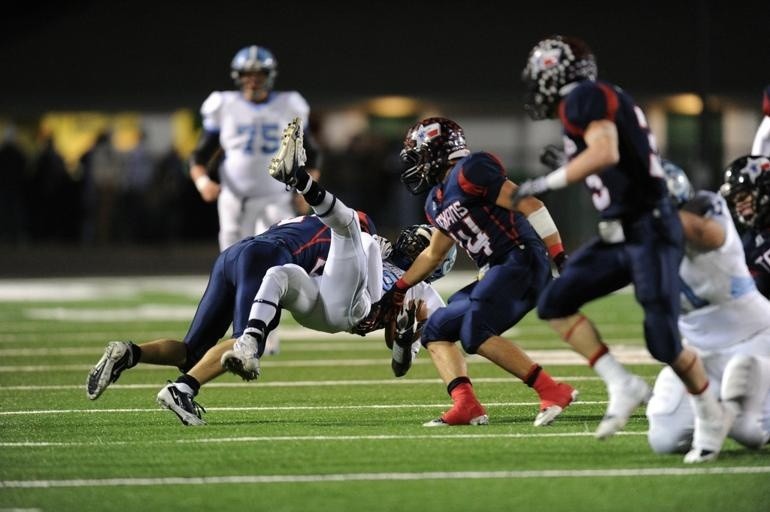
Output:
[643, 156, 769, 458]
[506, 30, 741, 465]
[719, 155, 769, 302]
[750, 80, 770, 160]
[0, 106, 428, 249]
[84, 206, 378, 402]
[184, 43, 313, 360]
[221, 116, 461, 386]
[380, 115, 580, 427]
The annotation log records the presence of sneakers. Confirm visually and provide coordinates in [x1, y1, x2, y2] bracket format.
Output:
[684, 400, 735, 465]
[268, 117, 308, 188]
[220, 333, 260, 381]
[533, 382, 579, 428]
[422, 399, 489, 427]
[157, 379, 207, 425]
[86, 341, 132, 401]
[597, 373, 649, 439]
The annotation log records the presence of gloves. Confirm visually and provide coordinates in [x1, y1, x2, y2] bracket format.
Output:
[510, 175, 548, 212]
[373, 233, 394, 261]
[375, 284, 406, 317]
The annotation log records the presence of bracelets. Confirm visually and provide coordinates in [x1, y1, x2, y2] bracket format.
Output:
[547, 244, 565, 258]
[393, 278, 412, 295]
[547, 163, 572, 196]
[190, 174, 211, 195]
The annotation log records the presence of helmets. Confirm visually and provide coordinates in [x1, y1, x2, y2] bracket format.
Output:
[400, 117, 471, 195]
[721, 155, 768, 233]
[517, 34, 600, 121]
[393, 221, 458, 283]
[230, 46, 278, 89]
[661, 158, 695, 209]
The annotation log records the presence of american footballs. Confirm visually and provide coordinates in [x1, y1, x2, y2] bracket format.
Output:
[399, 300, 427, 344]
